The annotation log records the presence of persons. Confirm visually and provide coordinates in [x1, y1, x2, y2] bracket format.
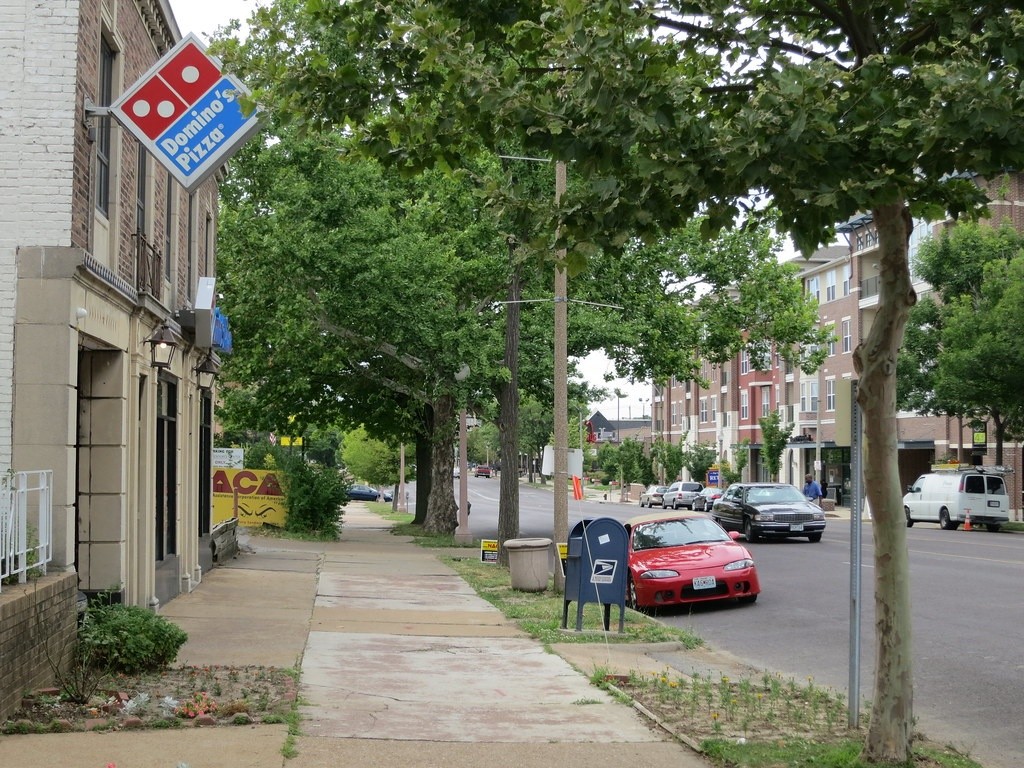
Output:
[820, 479, 828, 499]
[802, 474, 822, 506]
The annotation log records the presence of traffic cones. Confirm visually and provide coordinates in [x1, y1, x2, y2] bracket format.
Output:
[962, 508, 974, 531]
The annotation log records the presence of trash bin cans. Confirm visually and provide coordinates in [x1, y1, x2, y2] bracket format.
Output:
[562, 517, 630, 633]
[503, 537, 553, 593]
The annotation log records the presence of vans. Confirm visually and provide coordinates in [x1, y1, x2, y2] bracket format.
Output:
[661, 481, 705, 510]
[902, 462, 1014, 532]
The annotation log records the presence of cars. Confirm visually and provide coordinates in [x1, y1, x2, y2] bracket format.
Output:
[710, 482, 827, 543]
[638, 485, 671, 508]
[621, 508, 761, 617]
[474, 465, 490, 478]
[453, 466, 461, 479]
[691, 486, 727, 512]
[345, 483, 392, 502]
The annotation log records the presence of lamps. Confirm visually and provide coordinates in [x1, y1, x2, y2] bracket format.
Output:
[140, 315, 180, 377]
[191, 346, 219, 396]
